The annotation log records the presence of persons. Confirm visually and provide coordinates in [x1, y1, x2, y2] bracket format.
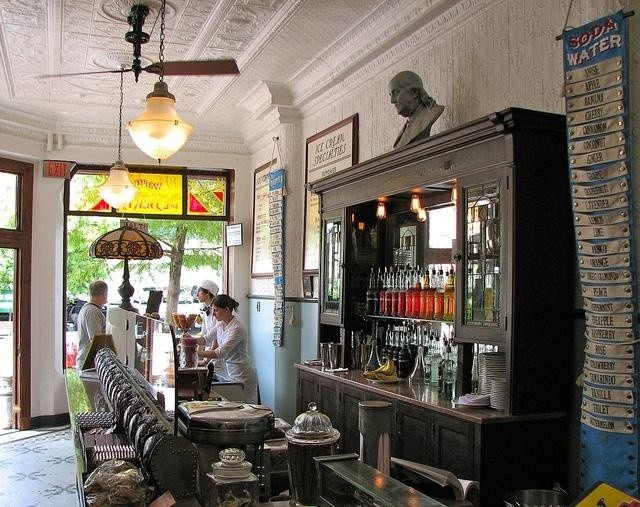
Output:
[385, 70, 446, 150]
[195, 280, 220, 367]
[75, 281, 108, 370]
[181, 293, 261, 407]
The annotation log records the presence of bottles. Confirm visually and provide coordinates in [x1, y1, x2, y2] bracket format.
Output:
[350, 318, 456, 399]
[367, 264, 457, 323]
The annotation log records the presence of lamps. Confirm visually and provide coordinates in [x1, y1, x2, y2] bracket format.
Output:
[126, 3, 195, 165]
[98, 66, 140, 211]
[86, 218, 164, 314]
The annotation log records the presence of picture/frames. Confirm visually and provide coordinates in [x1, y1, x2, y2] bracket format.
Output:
[302, 113, 360, 276]
[249, 158, 284, 280]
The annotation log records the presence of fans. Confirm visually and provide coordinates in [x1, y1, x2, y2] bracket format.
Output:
[31, 4, 240, 83]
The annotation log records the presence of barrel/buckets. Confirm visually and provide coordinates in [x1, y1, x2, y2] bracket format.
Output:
[500, 482, 572, 507]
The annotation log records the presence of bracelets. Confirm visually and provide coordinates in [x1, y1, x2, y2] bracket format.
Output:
[205, 360, 208, 365]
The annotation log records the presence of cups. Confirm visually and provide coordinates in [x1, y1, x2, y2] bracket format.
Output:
[319, 340, 342, 369]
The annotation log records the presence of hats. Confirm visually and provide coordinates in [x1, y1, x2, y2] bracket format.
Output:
[199, 278, 219, 296]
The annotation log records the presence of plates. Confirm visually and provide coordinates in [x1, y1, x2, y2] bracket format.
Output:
[478, 350, 505, 411]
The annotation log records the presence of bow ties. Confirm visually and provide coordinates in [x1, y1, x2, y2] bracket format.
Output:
[203, 306, 210, 315]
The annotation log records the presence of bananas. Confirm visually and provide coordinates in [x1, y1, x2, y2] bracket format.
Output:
[364, 358, 396, 382]
